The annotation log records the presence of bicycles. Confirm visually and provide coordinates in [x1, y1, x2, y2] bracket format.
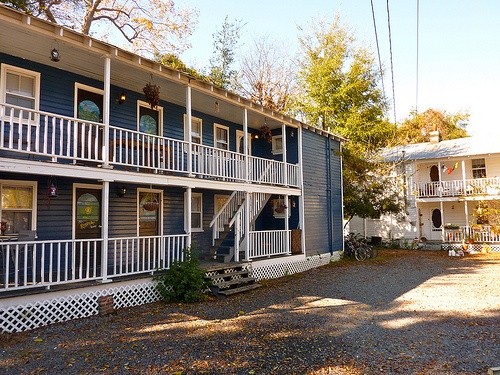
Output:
[344, 232, 379, 261]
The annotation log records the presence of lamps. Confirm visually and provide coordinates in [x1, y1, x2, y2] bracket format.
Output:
[48, 176, 57, 198]
[215, 101, 219, 113]
[120, 185, 127, 198]
[50, 49, 60, 62]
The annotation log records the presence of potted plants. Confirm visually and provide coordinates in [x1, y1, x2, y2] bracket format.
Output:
[140, 197, 160, 211]
